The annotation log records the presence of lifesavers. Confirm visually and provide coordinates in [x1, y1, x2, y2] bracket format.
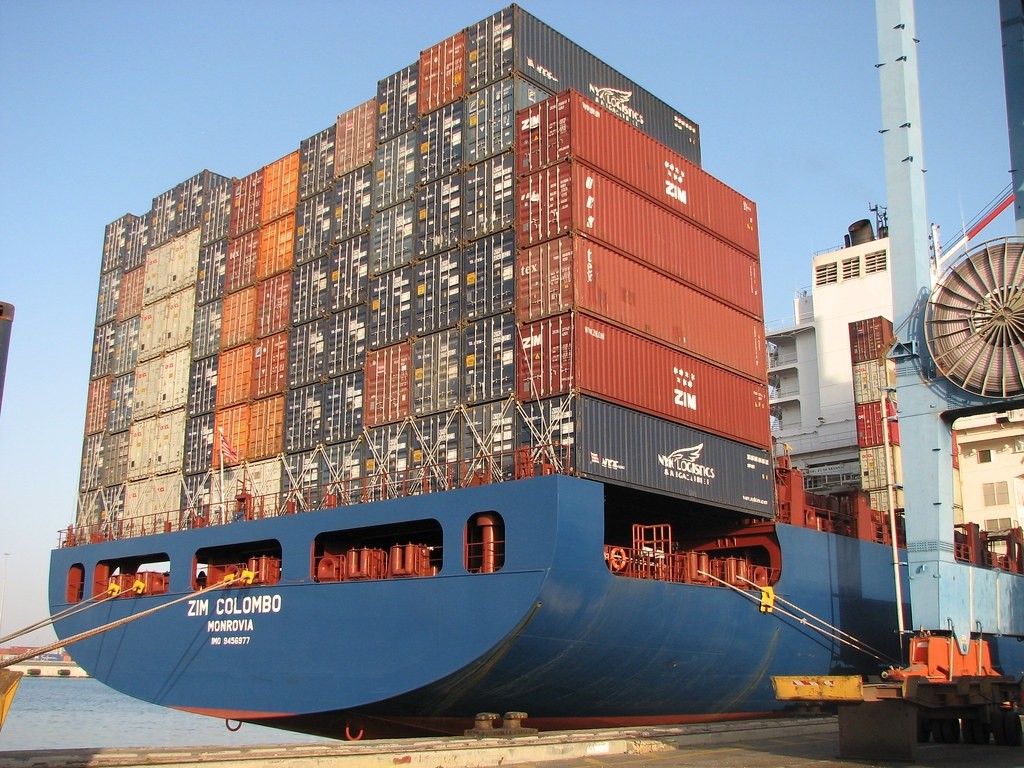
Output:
[609, 547, 627, 570]
